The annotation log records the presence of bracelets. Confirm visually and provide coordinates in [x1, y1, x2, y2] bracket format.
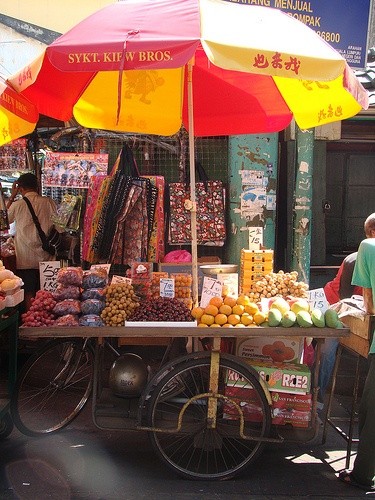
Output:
[9, 196, 14, 201]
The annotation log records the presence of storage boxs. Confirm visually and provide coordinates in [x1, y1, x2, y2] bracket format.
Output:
[223, 335, 312, 429]
[238, 247, 274, 295]
[337, 303, 375, 359]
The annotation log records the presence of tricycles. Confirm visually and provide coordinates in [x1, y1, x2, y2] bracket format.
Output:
[9, 335, 343, 482]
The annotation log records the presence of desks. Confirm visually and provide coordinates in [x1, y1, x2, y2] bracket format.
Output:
[322, 342, 369, 469]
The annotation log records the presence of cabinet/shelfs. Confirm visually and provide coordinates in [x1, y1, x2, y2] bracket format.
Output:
[0, 311, 20, 442]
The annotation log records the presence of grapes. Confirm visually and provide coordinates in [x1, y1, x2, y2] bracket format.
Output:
[22, 289, 56, 328]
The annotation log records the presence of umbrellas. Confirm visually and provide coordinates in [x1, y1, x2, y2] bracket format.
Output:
[6, 0, 371, 361]
[0, 57, 40, 148]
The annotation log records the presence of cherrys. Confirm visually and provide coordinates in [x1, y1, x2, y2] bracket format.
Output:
[127, 297, 192, 321]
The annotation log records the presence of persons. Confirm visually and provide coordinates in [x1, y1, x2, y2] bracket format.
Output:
[310, 251, 363, 455]
[339, 212, 375, 492]
[7, 171, 63, 360]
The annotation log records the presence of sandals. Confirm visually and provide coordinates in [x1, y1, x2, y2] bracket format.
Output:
[338, 468, 375, 491]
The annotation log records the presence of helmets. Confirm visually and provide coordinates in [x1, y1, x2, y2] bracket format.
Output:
[107, 353, 148, 398]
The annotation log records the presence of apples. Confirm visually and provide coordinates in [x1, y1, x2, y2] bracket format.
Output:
[0, 260, 22, 295]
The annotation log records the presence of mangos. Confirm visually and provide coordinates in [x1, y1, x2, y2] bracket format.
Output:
[261, 300, 343, 329]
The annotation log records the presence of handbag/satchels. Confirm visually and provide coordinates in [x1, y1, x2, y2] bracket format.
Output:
[80, 140, 228, 266]
[41, 227, 64, 257]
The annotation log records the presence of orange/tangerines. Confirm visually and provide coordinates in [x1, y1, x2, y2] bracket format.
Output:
[191, 295, 265, 328]
